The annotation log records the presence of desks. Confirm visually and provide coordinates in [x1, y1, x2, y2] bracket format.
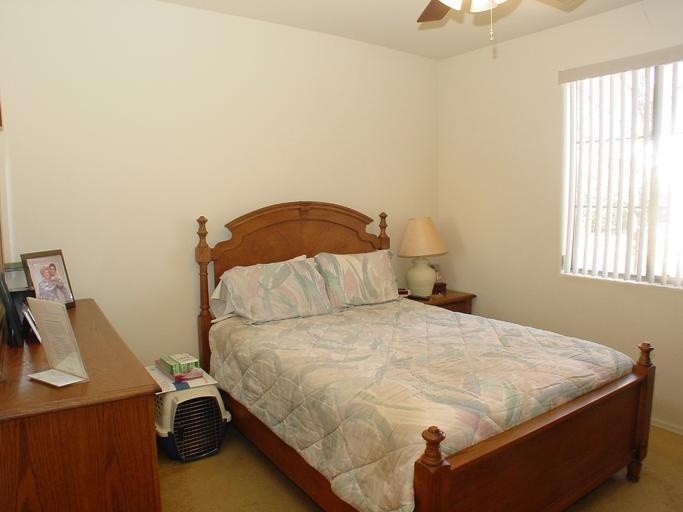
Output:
[0, 298, 161, 512]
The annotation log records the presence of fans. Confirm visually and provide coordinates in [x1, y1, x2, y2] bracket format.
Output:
[416, 0, 451, 22]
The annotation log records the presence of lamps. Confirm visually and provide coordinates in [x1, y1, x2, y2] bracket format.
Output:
[397, 217, 448, 299]
[439, 0, 507, 12]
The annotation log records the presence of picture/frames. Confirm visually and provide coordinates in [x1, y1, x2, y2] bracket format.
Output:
[20, 248, 75, 309]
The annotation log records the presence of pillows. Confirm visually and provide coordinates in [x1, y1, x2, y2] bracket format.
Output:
[219, 257, 332, 323]
[315, 249, 400, 309]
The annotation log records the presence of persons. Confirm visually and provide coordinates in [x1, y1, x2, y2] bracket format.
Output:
[46, 264, 69, 303]
[36, 266, 63, 304]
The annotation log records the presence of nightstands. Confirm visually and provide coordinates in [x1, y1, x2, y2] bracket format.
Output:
[403, 290, 477, 314]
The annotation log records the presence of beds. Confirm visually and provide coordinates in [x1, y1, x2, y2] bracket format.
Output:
[195, 200, 656, 511]
